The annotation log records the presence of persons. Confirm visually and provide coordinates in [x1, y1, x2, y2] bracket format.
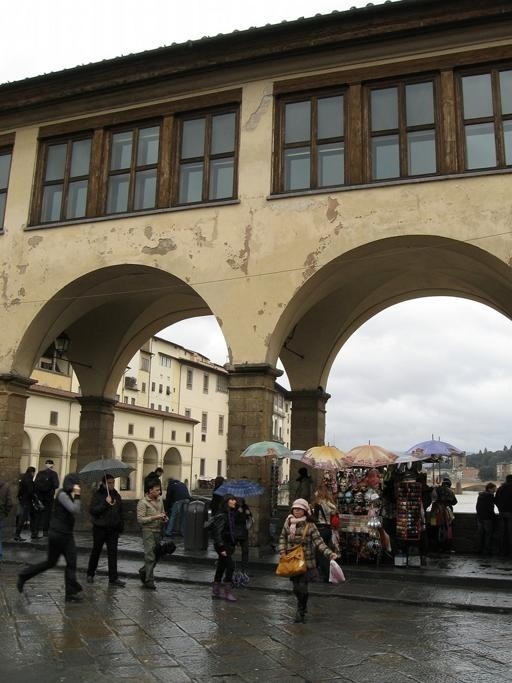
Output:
[87, 474, 126, 586]
[234, 497, 254, 577]
[166, 475, 192, 538]
[279, 499, 338, 624]
[211, 495, 236, 602]
[293, 467, 313, 504]
[476, 482, 497, 553]
[139, 480, 168, 591]
[211, 476, 226, 516]
[416, 473, 432, 510]
[495, 475, 512, 556]
[34, 461, 59, 537]
[16, 473, 84, 602]
[0, 479, 12, 524]
[15, 467, 40, 540]
[436, 478, 458, 513]
[143, 468, 164, 496]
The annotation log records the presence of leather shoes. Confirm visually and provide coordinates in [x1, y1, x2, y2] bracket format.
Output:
[110, 579, 125, 585]
[17, 573, 24, 594]
[65, 593, 83, 602]
[87, 574, 93, 582]
[145, 581, 157, 589]
[139, 570, 147, 582]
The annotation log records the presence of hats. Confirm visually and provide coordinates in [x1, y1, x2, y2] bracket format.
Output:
[291, 498, 310, 512]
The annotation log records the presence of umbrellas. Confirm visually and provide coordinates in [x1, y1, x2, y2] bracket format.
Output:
[405, 435, 465, 486]
[241, 441, 293, 459]
[290, 449, 308, 461]
[396, 452, 431, 463]
[78, 454, 138, 505]
[301, 442, 347, 470]
[215, 478, 266, 499]
[347, 440, 399, 469]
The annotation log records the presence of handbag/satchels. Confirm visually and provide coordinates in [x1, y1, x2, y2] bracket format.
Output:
[276, 523, 309, 577]
[309, 504, 331, 541]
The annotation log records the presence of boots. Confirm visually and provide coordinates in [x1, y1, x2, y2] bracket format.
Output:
[212, 582, 225, 599]
[295, 593, 308, 623]
[224, 582, 237, 601]
[295, 591, 308, 615]
